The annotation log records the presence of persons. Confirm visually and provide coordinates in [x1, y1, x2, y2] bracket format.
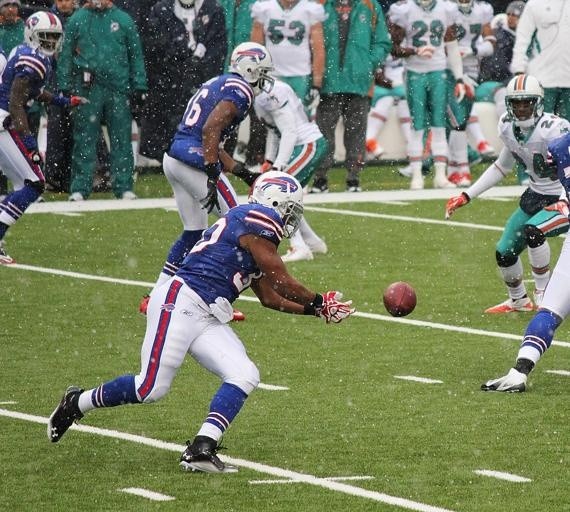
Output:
[0, 10, 90, 267]
[0, 0, 569, 203]
[46, 171, 356, 475]
[446, 74, 570, 314]
[139, 41, 275, 321]
[253, 76, 329, 262]
[480, 131, 570, 394]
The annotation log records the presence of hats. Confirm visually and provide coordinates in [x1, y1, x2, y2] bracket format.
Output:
[0, 0, 21, 8]
[507, 1, 524, 17]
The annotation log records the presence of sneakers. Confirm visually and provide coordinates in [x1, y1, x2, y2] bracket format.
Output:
[398, 145, 494, 189]
[47, 387, 82, 442]
[346, 179, 360, 191]
[485, 289, 544, 314]
[0, 247, 15, 263]
[279, 240, 327, 262]
[69, 192, 83, 201]
[177, 435, 239, 475]
[122, 191, 137, 200]
[231, 309, 246, 320]
[363, 144, 383, 162]
[308, 178, 327, 193]
[138, 295, 151, 314]
[482, 367, 528, 393]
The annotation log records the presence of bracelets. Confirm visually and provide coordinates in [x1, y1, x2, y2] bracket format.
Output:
[303, 303, 315, 315]
[313, 295, 323, 307]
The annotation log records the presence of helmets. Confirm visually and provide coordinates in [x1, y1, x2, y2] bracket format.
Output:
[504, 74, 547, 128]
[249, 170, 304, 239]
[229, 41, 276, 96]
[24, 11, 64, 57]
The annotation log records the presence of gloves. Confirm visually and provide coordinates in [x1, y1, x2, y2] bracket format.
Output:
[232, 162, 260, 184]
[52, 96, 89, 108]
[444, 194, 468, 218]
[416, 45, 435, 58]
[22, 134, 45, 164]
[454, 75, 479, 103]
[201, 163, 223, 215]
[303, 290, 356, 324]
[546, 201, 569, 218]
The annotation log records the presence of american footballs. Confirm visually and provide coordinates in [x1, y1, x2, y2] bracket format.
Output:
[383, 282, 416, 316]
